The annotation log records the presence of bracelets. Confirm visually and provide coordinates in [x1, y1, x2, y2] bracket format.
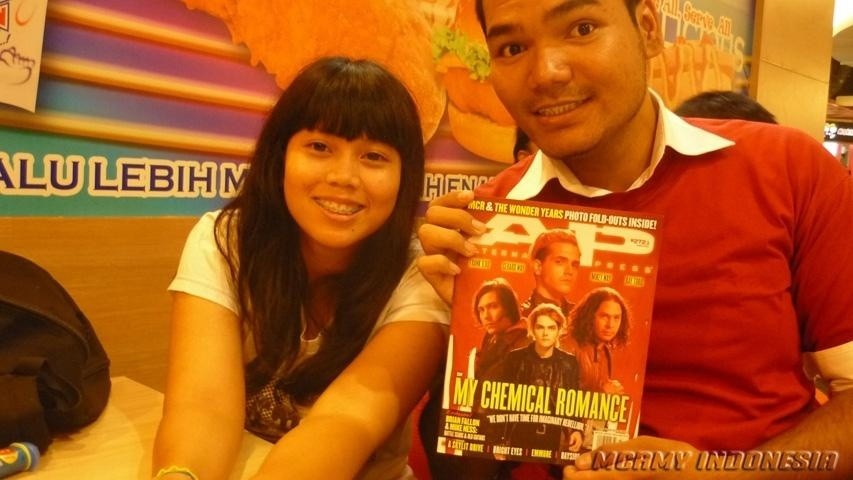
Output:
[153, 465, 198, 480]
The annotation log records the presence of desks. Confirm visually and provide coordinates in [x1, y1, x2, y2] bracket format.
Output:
[0, 375, 280, 480]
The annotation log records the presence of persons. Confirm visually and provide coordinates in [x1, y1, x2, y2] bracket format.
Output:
[412, 0, 851, 480]
[517, 228, 581, 322]
[558, 286, 632, 455]
[490, 302, 585, 461]
[469, 276, 523, 418]
[672, 90, 778, 125]
[147, 53, 460, 480]
[511, 125, 542, 165]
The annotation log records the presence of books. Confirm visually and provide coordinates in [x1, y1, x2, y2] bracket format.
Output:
[433, 193, 661, 475]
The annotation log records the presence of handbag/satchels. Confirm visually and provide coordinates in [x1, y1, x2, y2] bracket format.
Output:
[0, 250, 111, 455]
